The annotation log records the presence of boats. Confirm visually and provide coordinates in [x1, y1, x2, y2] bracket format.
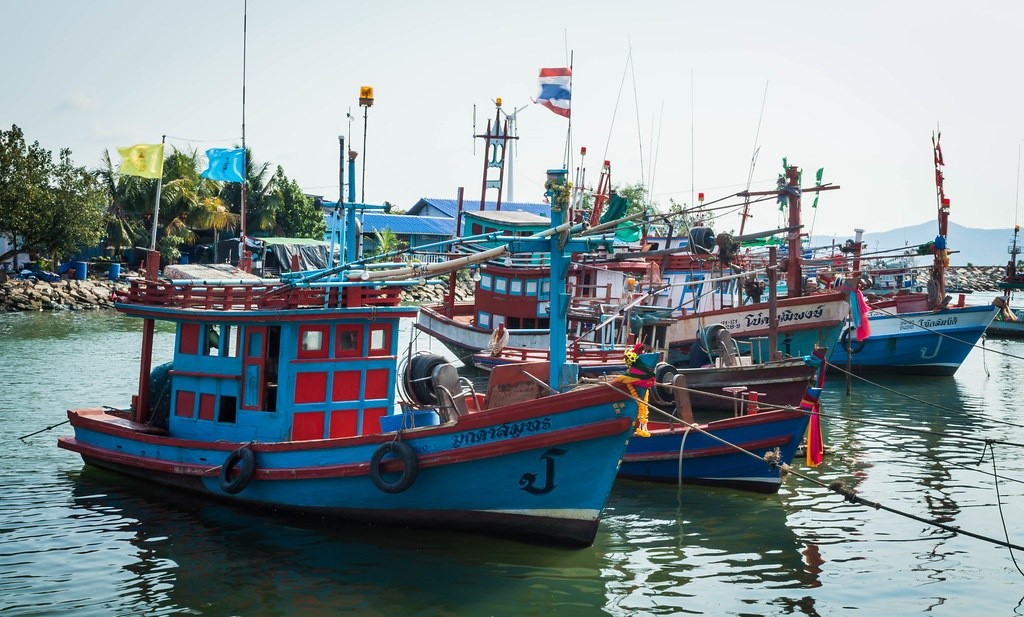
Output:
[54, 0, 1024, 550]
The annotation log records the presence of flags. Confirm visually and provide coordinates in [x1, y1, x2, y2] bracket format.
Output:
[777, 156, 824, 212]
[931, 131, 945, 198]
[199, 148, 246, 184]
[115, 142, 165, 179]
[530, 66, 571, 120]
